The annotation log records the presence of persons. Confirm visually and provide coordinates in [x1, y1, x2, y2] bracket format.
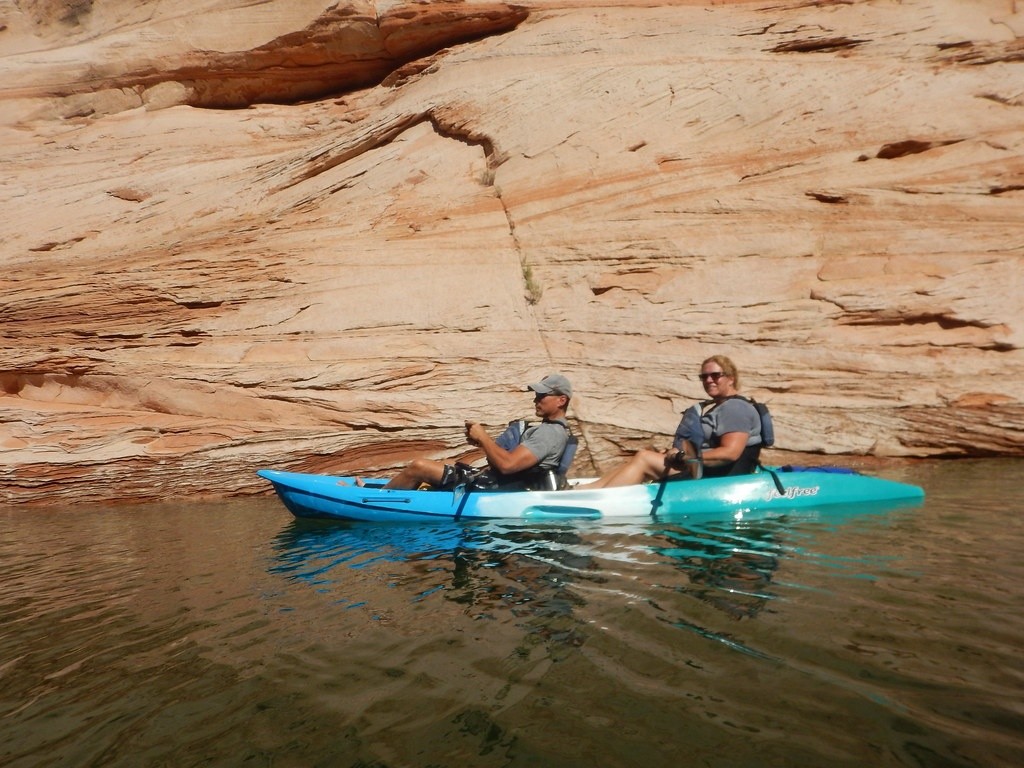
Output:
[562, 355, 762, 488]
[337, 374, 572, 490]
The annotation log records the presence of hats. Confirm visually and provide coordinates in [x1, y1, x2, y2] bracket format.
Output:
[527, 375, 572, 399]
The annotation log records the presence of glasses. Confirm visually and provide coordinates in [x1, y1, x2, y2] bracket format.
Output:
[699, 372, 726, 381]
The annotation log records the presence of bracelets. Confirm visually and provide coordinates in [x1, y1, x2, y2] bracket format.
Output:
[676, 450, 685, 462]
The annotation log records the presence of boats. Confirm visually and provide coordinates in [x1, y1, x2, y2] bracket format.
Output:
[257, 464, 926, 524]
